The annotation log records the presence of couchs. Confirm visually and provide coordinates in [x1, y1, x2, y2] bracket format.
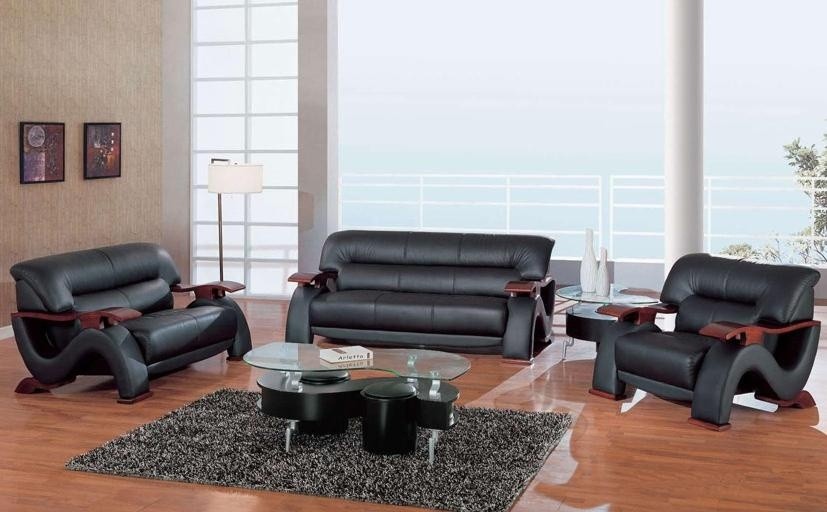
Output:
[284, 230, 556, 367]
[9, 242, 251, 403]
[588, 253, 820, 432]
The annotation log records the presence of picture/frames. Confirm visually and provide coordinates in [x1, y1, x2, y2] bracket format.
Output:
[18, 120, 121, 184]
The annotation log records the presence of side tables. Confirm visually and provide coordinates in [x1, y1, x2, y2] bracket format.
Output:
[556, 284, 658, 360]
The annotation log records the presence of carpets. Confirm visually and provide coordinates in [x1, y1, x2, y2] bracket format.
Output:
[63, 387, 577, 511]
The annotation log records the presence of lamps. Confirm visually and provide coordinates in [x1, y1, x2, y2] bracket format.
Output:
[208, 157, 263, 281]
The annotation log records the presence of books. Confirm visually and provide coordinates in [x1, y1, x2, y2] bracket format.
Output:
[319, 345, 372, 363]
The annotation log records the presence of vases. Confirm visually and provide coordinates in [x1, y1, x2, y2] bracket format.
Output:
[579, 228, 609, 297]
